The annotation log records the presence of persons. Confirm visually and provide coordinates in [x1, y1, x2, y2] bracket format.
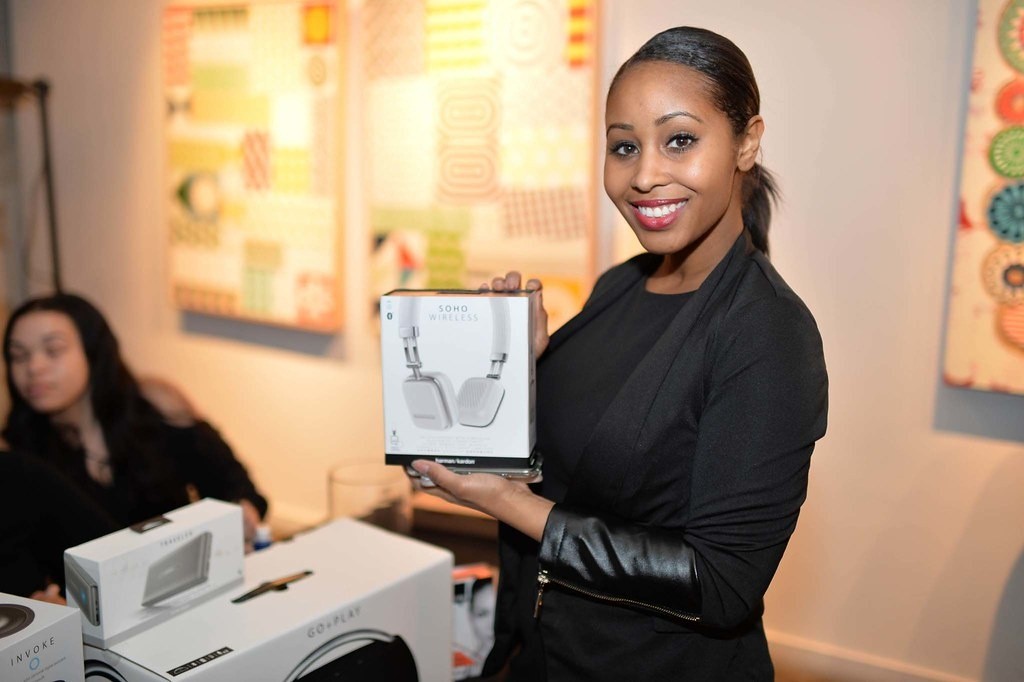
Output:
[2, 289, 269, 606]
[409, 24, 830, 682]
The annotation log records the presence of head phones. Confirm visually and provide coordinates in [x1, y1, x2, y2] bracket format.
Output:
[398, 296, 510, 431]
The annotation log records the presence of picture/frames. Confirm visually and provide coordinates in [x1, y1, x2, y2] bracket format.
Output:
[939, 0, 1022, 398]
[156, 0, 617, 368]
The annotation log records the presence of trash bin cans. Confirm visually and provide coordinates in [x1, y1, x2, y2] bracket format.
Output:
[327, 455, 414, 537]
